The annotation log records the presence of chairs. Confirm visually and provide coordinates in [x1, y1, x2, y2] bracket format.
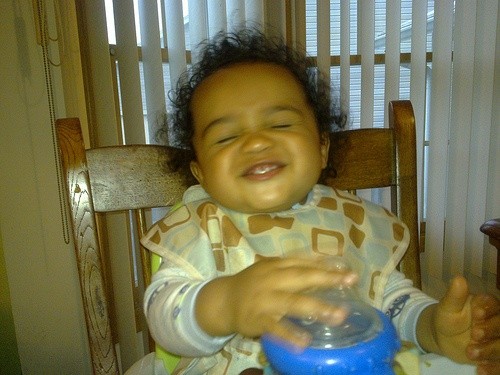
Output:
[54, 98, 422, 375]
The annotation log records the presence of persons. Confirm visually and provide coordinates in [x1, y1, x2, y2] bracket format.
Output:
[122, 24, 500, 374]
